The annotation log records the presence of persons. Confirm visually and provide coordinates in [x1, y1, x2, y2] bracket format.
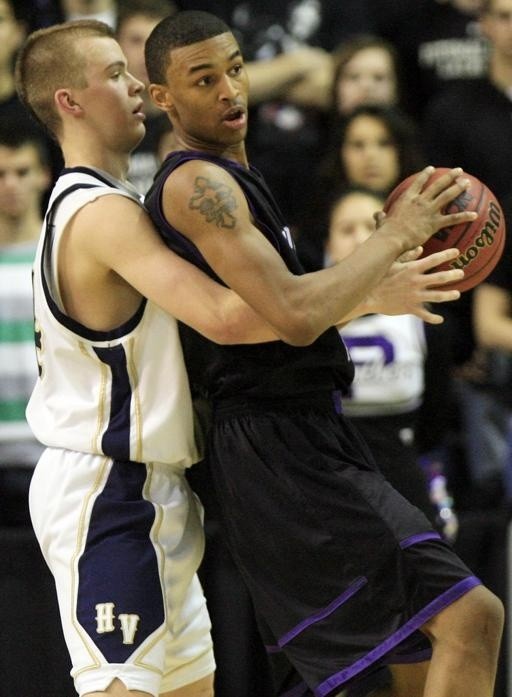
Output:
[0, 1, 507, 697]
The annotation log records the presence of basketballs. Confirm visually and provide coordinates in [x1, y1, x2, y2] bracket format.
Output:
[383, 168, 505, 293]
